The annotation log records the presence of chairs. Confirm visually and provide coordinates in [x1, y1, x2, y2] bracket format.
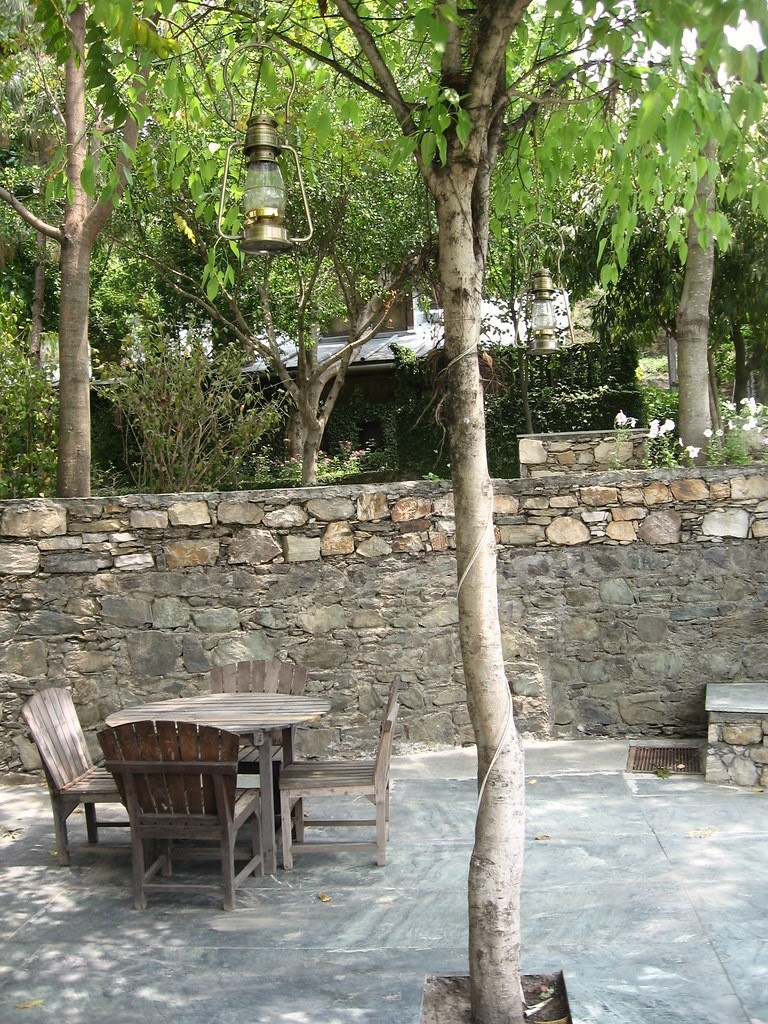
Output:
[95, 719, 264, 912]
[21, 687, 155, 866]
[279, 674, 399, 870]
[209, 659, 308, 843]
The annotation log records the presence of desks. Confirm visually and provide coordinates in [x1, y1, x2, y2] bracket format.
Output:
[105, 693, 330, 877]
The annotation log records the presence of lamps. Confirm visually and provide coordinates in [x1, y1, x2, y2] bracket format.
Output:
[217, 3, 313, 256]
[517, 109, 577, 356]
